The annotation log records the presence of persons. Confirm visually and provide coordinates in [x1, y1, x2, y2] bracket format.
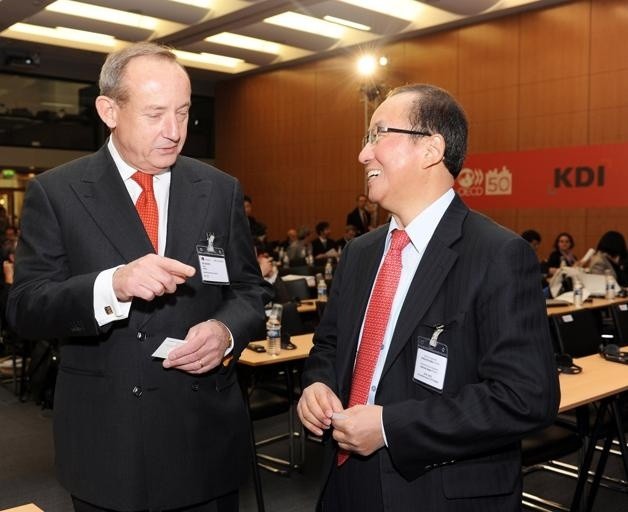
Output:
[7, 39, 271, 511]
[294, 84, 562, 511]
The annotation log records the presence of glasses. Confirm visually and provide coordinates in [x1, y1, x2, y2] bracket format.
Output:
[360, 123, 433, 149]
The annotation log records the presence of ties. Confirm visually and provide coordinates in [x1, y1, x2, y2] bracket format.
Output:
[335, 227, 412, 468]
[130, 169, 160, 258]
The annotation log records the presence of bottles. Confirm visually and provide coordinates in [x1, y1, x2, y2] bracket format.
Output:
[603, 270, 615, 299]
[266, 312, 281, 358]
[276, 245, 316, 267]
[318, 280, 328, 301]
[571, 275, 583, 308]
[324, 260, 334, 281]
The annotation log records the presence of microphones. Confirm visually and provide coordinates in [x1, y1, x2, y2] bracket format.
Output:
[281, 296, 313, 349]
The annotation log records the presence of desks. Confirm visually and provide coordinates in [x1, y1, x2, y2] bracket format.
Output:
[236, 224, 341, 511]
[523, 224, 627, 512]
[2, 227, 59, 409]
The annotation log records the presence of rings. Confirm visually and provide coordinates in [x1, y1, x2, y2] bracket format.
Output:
[198, 359, 205, 368]
